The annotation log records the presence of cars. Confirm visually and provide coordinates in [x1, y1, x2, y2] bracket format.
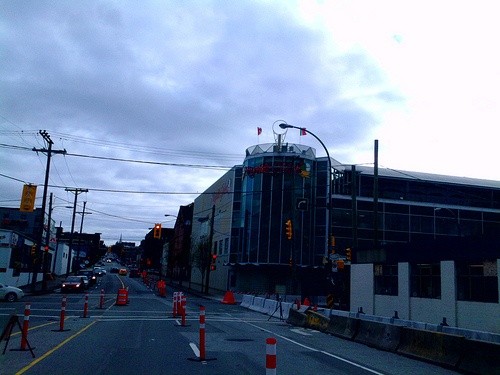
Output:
[61, 276, 85, 293]
[93, 256, 127, 277]
[77, 276, 90, 288]
[0, 282, 25, 302]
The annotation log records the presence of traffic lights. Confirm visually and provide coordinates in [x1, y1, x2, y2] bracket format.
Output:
[154, 224, 161, 239]
[210, 265, 216, 271]
[346, 247, 352, 261]
[31, 245, 36, 256]
[285, 220, 292, 240]
[212, 255, 216, 264]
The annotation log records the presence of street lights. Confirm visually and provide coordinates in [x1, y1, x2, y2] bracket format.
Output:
[41, 205, 75, 295]
[278, 123, 331, 265]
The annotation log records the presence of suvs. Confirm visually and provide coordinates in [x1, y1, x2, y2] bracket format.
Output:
[77, 269, 99, 284]
